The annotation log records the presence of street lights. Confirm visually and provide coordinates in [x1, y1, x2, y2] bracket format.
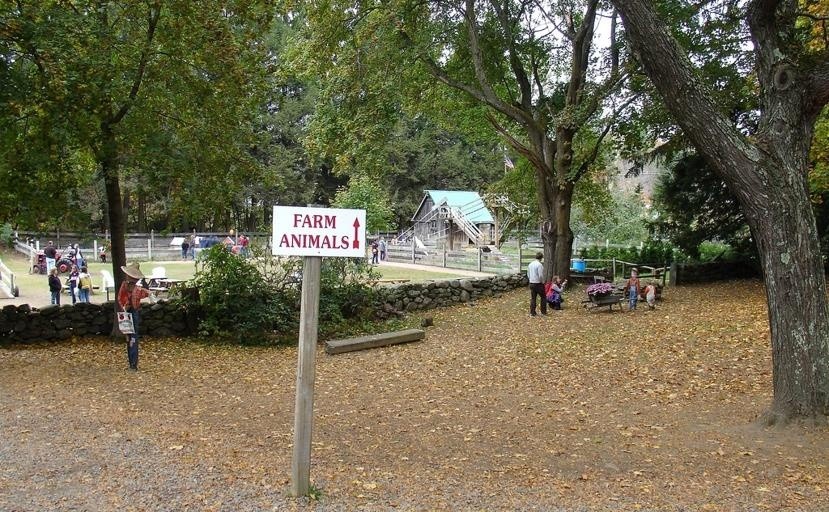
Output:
[230, 229, 238, 245]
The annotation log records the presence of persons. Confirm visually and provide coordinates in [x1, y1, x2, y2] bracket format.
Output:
[526, 252, 546, 316]
[623, 267, 640, 310]
[71, 243, 85, 271]
[372, 234, 398, 265]
[67, 265, 80, 304]
[114, 262, 157, 368]
[47, 265, 61, 305]
[74, 267, 93, 303]
[44, 241, 57, 275]
[182, 234, 249, 260]
[644, 280, 657, 309]
[546, 274, 568, 309]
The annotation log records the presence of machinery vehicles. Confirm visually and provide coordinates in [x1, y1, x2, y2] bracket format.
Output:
[29, 244, 88, 276]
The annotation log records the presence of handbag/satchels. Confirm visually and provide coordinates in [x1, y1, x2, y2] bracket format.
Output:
[78, 276, 82, 288]
[117, 311, 135, 334]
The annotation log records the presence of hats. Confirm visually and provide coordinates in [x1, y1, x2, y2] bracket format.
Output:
[121, 260, 145, 279]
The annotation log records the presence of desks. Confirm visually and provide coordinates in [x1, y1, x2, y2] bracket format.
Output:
[61, 276, 188, 303]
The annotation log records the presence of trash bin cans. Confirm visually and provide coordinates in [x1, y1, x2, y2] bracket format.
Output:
[572, 258, 585, 273]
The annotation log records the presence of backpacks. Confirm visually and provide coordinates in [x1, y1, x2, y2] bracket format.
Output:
[544, 282, 551, 295]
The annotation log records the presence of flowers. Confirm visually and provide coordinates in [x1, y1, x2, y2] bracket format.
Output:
[587, 282, 613, 296]
